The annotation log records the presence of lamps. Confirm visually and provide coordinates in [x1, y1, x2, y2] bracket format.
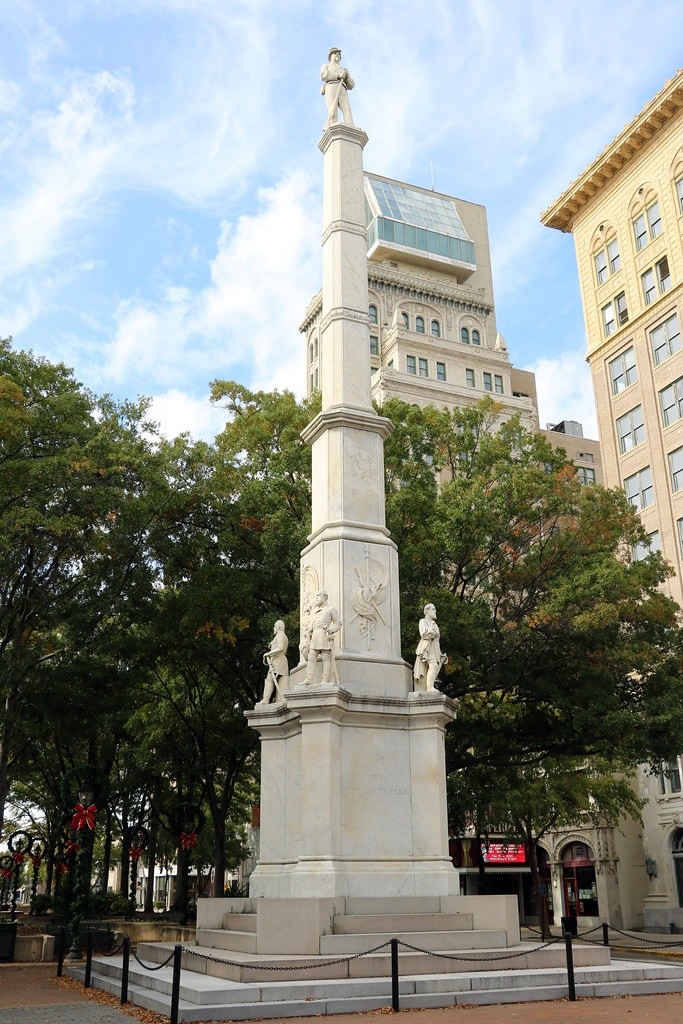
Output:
[646, 856, 657, 881]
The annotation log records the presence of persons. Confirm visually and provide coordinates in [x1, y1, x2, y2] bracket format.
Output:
[320, 47, 361, 132]
[299, 590, 343, 686]
[256, 619, 289, 705]
[414, 604, 448, 692]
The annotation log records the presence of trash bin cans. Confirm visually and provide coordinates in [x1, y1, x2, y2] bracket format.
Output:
[561, 917, 577, 936]
[0, 922, 18, 962]
